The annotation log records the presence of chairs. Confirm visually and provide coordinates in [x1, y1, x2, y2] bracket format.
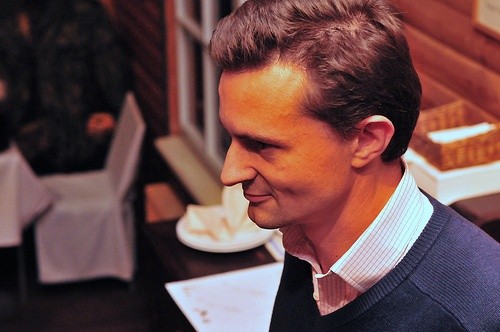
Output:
[34, 91, 145, 287]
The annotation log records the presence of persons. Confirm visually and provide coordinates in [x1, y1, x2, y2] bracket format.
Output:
[1, 1, 129, 181]
[207, 0, 499, 332]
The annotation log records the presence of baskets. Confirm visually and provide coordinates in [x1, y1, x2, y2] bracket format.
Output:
[411, 99, 500, 173]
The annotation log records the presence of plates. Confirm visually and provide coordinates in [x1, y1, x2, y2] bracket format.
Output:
[177, 210, 276, 254]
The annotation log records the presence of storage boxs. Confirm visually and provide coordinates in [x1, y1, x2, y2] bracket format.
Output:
[409, 98, 499, 172]
